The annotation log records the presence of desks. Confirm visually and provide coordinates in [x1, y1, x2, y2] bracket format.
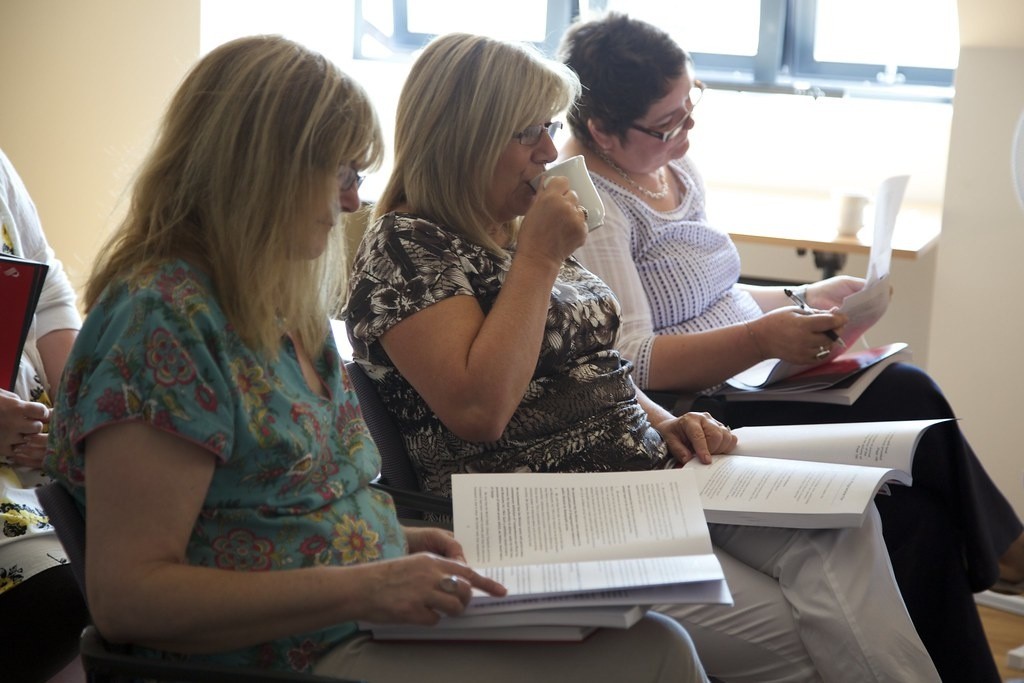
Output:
[359, 170, 941, 286]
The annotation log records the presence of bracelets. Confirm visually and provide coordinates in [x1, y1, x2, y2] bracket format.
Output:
[792, 285, 808, 306]
[745, 322, 766, 361]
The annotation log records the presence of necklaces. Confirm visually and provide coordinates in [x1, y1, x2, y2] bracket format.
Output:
[586, 145, 669, 200]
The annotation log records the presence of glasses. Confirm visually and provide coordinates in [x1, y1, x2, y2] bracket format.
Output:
[630, 79, 707, 143]
[509, 121, 564, 147]
[334, 162, 366, 193]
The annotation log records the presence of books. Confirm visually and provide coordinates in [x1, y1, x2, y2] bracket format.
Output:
[714, 341, 915, 407]
[680, 419, 962, 530]
[0, 248, 50, 394]
[357, 467, 735, 645]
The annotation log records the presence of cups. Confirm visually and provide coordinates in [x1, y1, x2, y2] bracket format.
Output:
[516, 155, 606, 232]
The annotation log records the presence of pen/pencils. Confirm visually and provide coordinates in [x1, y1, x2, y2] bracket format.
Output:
[784, 287, 849, 348]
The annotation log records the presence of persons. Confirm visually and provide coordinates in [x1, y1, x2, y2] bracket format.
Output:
[41, 33, 713, 682]
[344, 34, 944, 682]
[0, 145, 94, 683]
[544, 13, 1024, 683]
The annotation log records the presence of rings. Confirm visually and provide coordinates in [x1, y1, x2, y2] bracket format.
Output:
[576, 206, 589, 221]
[816, 346, 831, 360]
[722, 425, 732, 434]
[439, 575, 459, 592]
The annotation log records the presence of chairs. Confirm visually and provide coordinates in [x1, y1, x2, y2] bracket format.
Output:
[29, 363, 452, 682]
